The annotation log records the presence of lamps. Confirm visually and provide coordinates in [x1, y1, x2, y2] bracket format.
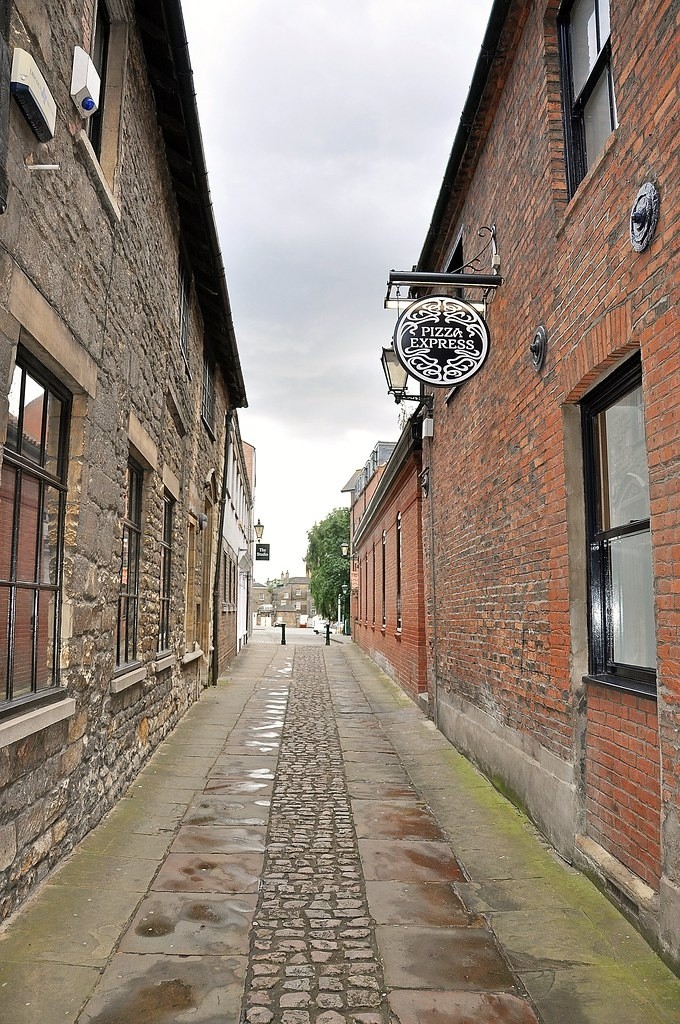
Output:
[253, 518, 265, 539]
[380, 336, 433, 417]
[340, 538, 350, 557]
